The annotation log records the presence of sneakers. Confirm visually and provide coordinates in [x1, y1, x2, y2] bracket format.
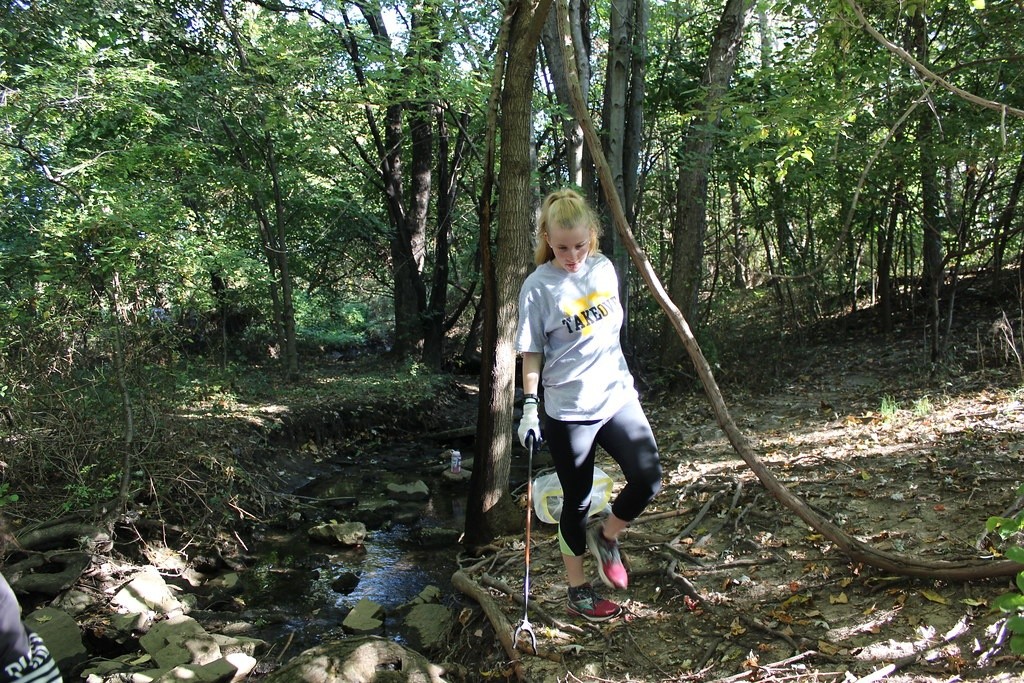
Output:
[586, 522, 628, 591]
[566, 582, 623, 623]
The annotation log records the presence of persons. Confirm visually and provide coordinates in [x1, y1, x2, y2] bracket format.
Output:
[0, 564, 63, 683]
[516, 189, 663, 623]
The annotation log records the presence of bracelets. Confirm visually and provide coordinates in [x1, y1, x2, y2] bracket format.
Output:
[522, 394, 540, 404]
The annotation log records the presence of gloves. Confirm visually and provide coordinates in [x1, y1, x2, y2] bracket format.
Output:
[517, 403, 543, 455]
